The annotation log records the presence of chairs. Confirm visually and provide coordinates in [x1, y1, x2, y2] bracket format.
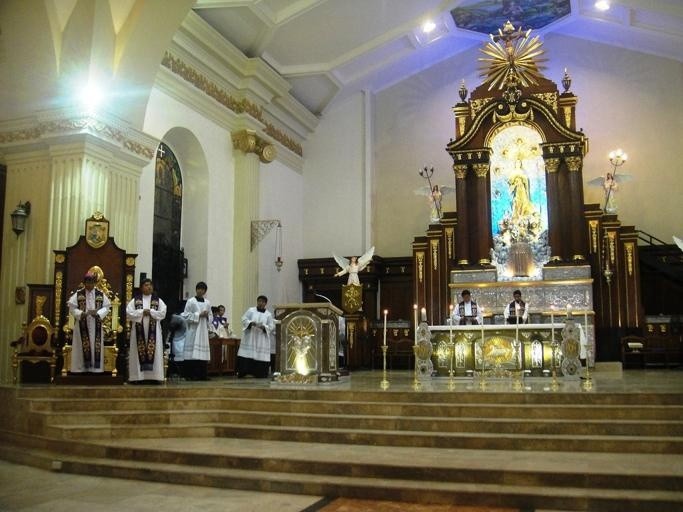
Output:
[60, 266, 123, 381]
[9, 314, 58, 385]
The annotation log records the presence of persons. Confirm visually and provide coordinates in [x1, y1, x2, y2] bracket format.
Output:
[427, 185, 442, 221]
[235, 295, 274, 378]
[217, 305, 231, 337]
[211, 303, 227, 362]
[333, 255, 370, 312]
[507, 158, 534, 220]
[183, 282, 212, 376]
[67, 271, 110, 373]
[599, 173, 618, 212]
[503, 290, 529, 324]
[125, 278, 167, 380]
[452, 290, 482, 324]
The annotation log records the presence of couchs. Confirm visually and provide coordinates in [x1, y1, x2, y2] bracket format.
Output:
[619, 334, 683, 369]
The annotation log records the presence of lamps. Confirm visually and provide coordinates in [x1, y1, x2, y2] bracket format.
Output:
[273, 222, 284, 273]
[600, 149, 627, 214]
[9, 200, 31, 241]
[416, 163, 443, 221]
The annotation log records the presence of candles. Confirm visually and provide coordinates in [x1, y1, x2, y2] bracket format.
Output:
[550, 304, 555, 342]
[515, 305, 519, 343]
[448, 305, 452, 343]
[584, 309, 588, 345]
[413, 304, 417, 346]
[382, 309, 387, 345]
[480, 306, 484, 344]
